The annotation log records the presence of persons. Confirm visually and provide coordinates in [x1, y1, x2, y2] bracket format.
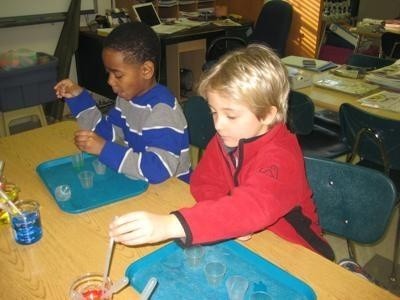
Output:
[53, 19, 192, 186]
[108, 40, 337, 263]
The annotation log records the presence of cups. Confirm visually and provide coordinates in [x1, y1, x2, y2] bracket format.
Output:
[203, 261, 226, 289]
[8, 199, 42, 243]
[72, 151, 85, 170]
[69, 272, 113, 300]
[183, 244, 204, 267]
[77, 170, 94, 189]
[226, 275, 248, 300]
[250, 291, 273, 300]
[92, 159, 106, 173]
[0, 181, 20, 227]
[55, 184, 71, 201]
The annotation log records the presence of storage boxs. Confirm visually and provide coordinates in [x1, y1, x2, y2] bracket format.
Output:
[0, 51, 58, 111]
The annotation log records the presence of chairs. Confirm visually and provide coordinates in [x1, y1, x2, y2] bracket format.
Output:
[285, 91, 350, 159]
[183, 96, 217, 167]
[203, 0, 293, 74]
[348, 54, 396, 72]
[338, 102, 400, 192]
[302, 154, 397, 271]
[381, 32, 400, 60]
[318, 21, 359, 65]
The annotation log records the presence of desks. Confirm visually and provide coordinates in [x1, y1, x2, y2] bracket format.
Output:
[75, 14, 254, 103]
[1, 120, 400, 300]
[279, 54, 400, 121]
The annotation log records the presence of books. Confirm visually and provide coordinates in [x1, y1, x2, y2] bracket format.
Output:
[279, 48, 400, 115]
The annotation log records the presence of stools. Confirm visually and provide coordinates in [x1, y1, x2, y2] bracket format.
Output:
[0, 104, 47, 141]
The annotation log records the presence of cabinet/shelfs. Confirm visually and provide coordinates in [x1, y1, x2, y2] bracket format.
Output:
[115, 0, 216, 23]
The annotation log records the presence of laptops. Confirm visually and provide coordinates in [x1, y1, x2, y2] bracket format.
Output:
[132, 2, 184, 35]
